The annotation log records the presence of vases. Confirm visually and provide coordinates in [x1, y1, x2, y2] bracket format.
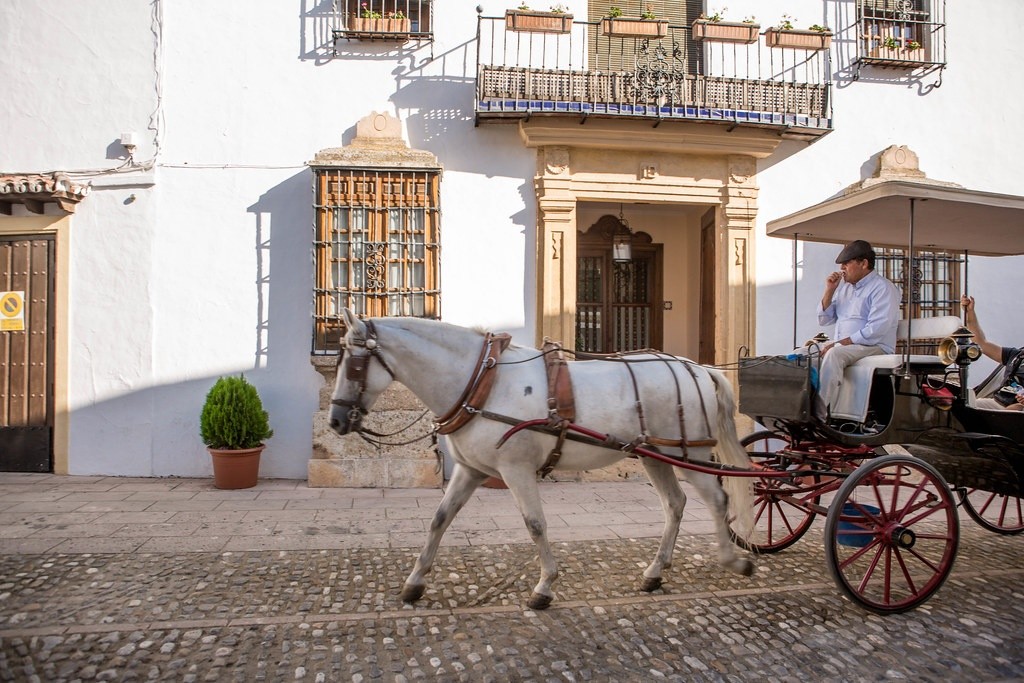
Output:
[600, 14, 670, 39]
[872, 46, 925, 63]
[351, 11, 412, 38]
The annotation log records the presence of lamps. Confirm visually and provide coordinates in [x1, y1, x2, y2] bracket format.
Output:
[599, 202, 634, 274]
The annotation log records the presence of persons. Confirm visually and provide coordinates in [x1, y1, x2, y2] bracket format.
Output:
[788, 240, 900, 424]
[959, 294, 1024, 411]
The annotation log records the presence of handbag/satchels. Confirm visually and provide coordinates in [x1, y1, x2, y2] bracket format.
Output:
[993, 383, 1017, 407]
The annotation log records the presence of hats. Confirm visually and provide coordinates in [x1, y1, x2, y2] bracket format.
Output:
[836, 240, 876, 263]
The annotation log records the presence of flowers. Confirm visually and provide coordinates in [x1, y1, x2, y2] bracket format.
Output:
[700, 7, 757, 23]
[904, 39, 920, 49]
[779, 13, 834, 30]
[519, 2, 569, 12]
[607, 4, 656, 20]
[356, 2, 404, 19]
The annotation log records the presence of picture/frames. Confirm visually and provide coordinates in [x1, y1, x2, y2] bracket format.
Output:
[692, 18, 760, 45]
[765, 27, 833, 51]
[503, 9, 574, 34]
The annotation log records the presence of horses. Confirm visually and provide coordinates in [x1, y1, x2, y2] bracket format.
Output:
[328, 307, 754, 609]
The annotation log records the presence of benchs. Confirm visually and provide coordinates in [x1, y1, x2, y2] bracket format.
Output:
[824, 315, 965, 426]
[974, 345, 1024, 417]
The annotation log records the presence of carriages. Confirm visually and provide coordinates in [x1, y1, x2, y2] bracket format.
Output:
[327, 145, 1024, 616]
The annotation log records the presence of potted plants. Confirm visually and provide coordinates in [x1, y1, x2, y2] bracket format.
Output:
[199, 372, 274, 490]
